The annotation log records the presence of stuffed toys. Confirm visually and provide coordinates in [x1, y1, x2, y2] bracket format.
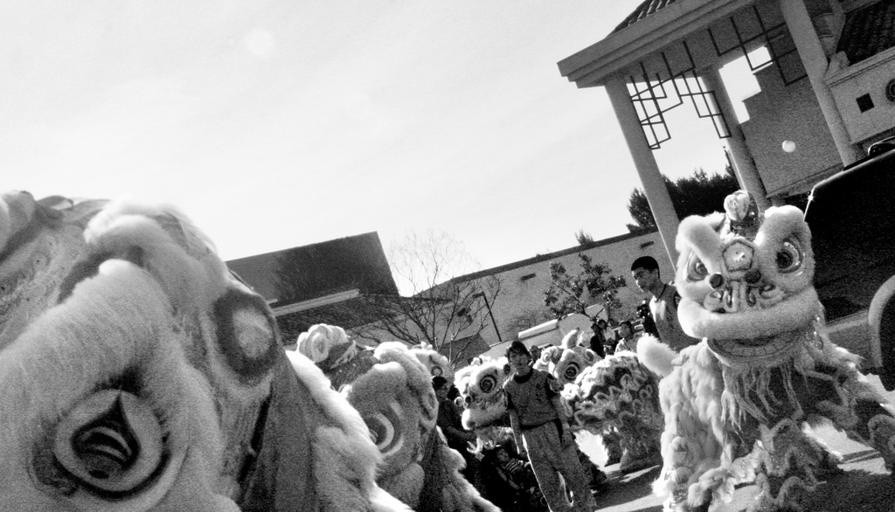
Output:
[648, 189, 895, 512]
[1, 189, 419, 512]
[294, 322, 502, 512]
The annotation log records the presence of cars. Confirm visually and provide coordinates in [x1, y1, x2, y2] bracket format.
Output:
[804, 136, 895, 390]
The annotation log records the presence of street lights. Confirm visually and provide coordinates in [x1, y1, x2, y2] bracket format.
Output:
[472, 291, 502, 342]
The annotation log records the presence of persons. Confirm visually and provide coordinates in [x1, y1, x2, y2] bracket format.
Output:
[432, 255, 702, 512]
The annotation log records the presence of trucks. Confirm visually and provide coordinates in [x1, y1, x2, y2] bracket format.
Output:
[518, 303, 611, 351]
